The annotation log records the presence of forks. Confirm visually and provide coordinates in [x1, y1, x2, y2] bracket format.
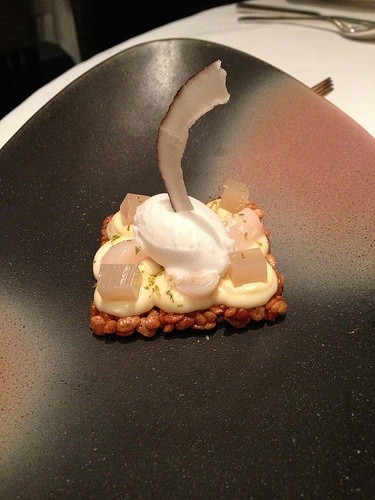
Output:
[309, 77, 335, 99]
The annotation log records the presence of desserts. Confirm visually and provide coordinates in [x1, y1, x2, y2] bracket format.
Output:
[89, 59, 285, 338]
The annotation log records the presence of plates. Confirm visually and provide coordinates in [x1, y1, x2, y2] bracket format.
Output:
[1, 35, 375, 499]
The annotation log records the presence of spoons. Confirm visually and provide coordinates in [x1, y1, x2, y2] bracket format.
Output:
[235, 3, 375, 39]
